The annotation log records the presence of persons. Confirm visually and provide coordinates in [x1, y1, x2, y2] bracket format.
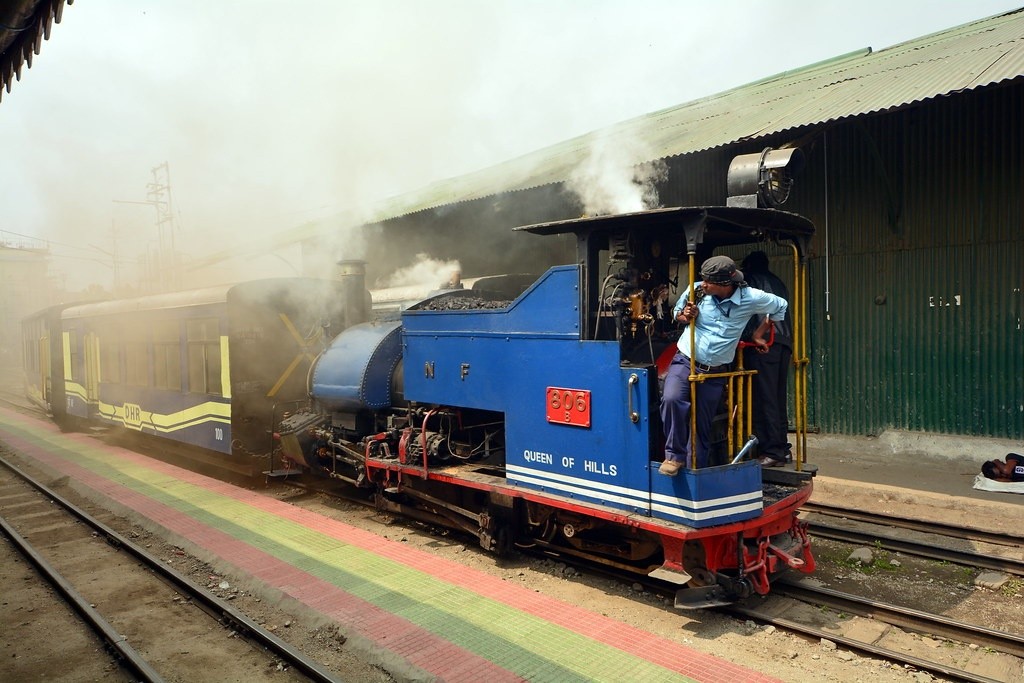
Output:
[625, 269, 672, 365]
[981, 452, 1024, 482]
[738, 249, 792, 467]
[659, 255, 787, 477]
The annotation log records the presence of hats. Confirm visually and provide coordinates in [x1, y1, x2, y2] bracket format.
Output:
[700, 255, 745, 286]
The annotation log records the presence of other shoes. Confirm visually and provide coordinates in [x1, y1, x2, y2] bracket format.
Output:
[782, 452, 792, 463]
[761, 454, 784, 467]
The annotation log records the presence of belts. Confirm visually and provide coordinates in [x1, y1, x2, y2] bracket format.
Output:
[678, 350, 727, 372]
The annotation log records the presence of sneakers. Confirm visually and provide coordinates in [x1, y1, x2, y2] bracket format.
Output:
[659, 459, 682, 476]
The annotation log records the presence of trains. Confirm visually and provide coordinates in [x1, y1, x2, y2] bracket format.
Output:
[21, 146, 819, 611]
